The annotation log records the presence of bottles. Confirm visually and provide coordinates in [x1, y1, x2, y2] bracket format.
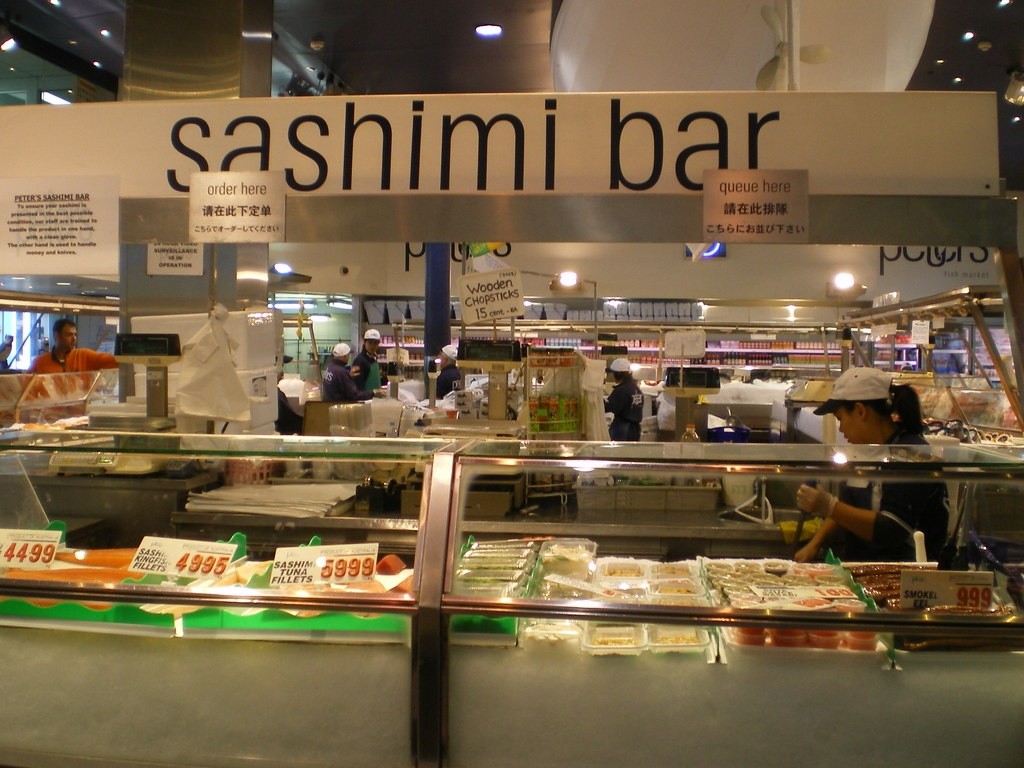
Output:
[368, 330, 912, 366]
[386, 422, 398, 438]
[398, 405, 431, 437]
[681, 423, 700, 443]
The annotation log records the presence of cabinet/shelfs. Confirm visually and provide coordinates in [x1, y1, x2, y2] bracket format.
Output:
[350, 292, 920, 372]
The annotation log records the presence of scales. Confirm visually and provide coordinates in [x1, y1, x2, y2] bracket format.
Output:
[665, 365, 721, 443]
[87, 334, 183, 429]
[359, 361, 405, 405]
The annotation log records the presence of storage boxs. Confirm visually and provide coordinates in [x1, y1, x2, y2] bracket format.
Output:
[572, 470, 722, 511]
[131, 310, 279, 435]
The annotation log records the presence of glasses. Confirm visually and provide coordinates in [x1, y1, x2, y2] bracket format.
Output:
[367, 340, 379, 344]
[438, 354, 441, 357]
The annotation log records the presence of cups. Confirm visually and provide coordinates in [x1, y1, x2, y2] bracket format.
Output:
[446, 408, 460, 423]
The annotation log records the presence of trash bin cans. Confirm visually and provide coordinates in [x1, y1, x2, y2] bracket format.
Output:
[707, 425, 752, 443]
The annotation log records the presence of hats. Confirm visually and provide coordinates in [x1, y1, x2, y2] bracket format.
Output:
[812, 366, 894, 416]
[276, 355, 294, 363]
[364, 329, 381, 343]
[604, 358, 632, 373]
[333, 343, 352, 357]
[436, 344, 458, 362]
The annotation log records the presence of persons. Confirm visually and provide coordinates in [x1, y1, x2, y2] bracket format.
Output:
[794, 368, 949, 563]
[323, 329, 387, 401]
[603, 358, 644, 441]
[274, 355, 304, 435]
[436, 345, 461, 399]
[0, 319, 119, 374]
